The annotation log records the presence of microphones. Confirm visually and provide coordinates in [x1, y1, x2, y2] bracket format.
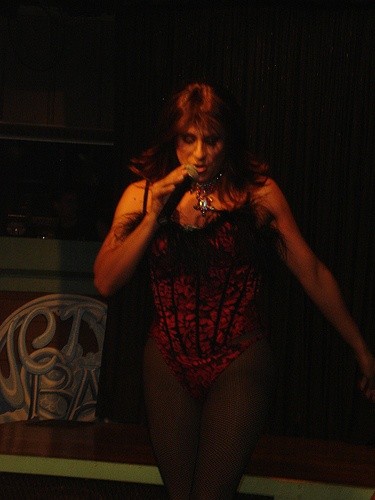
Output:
[155, 164, 200, 232]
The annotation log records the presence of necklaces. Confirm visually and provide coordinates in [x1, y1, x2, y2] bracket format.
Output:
[187, 172, 222, 219]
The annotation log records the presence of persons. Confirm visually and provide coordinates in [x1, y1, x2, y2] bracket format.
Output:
[92, 82, 375, 500]
